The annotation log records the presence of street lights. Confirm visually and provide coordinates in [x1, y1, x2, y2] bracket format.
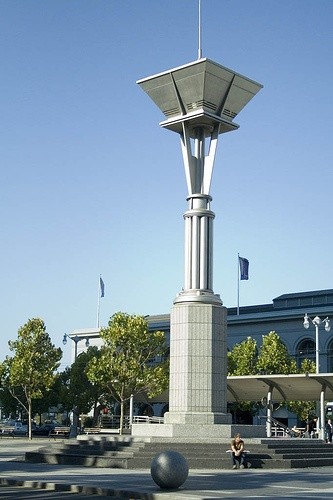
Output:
[303, 312, 331, 439]
[62, 332, 90, 437]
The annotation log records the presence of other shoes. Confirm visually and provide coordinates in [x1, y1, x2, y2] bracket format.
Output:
[239, 463, 244, 469]
[232, 465, 237, 468]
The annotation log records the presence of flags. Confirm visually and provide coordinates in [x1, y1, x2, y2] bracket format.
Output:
[100, 277, 104, 297]
[239, 256, 249, 280]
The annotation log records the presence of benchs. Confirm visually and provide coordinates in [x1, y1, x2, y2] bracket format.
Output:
[48, 426, 101, 441]
[0, 425, 15, 439]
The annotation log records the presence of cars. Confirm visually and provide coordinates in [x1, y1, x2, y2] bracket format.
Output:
[11, 420, 59, 435]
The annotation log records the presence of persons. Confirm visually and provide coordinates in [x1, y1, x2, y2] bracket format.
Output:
[309, 418, 318, 438]
[230, 433, 245, 469]
[326, 419, 332, 444]
